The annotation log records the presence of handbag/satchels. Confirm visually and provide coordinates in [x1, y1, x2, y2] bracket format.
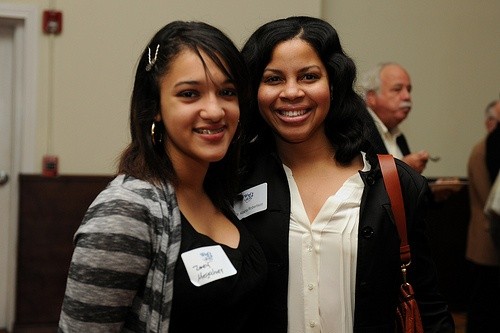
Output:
[397, 283, 424, 333]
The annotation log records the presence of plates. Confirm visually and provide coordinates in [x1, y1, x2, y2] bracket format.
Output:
[430, 183, 468, 192]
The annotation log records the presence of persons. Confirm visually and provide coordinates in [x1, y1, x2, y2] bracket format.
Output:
[359, 60, 432, 175]
[227, 16, 455, 333]
[465, 100, 500, 333]
[58, 20, 275, 333]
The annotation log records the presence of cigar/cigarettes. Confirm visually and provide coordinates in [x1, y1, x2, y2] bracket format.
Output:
[429, 153, 441, 162]
